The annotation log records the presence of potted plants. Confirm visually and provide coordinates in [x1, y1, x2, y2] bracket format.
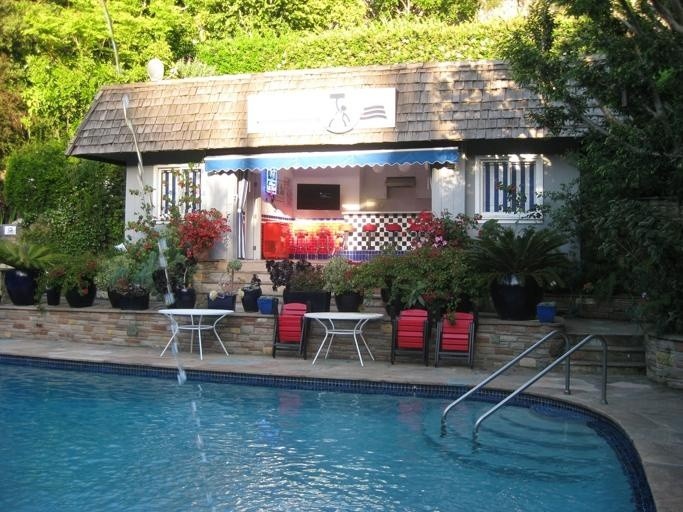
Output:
[0, 240, 50, 306]
[500, 11, 682, 394]
[45, 259, 63, 306]
[466, 225, 566, 322]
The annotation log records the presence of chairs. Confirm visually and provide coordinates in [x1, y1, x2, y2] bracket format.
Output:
[390, 309, 430, 367]
[271, 297, 311, 361]
[435, 311, 477, 369]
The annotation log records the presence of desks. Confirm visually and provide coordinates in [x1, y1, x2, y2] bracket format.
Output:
[156, 309, 234, 361]
[304, 311, 385, 368]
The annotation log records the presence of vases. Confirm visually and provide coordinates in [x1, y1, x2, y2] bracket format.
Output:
[64, 276, 97, 307]
[207, 285, 278, 315]
[106, 287, 197, 309]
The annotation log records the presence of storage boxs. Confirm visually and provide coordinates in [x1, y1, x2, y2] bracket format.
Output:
[537, 302, 558, 325]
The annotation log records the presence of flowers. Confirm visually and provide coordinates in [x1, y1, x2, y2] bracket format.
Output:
[96, 207, 229, 292]
[278, 243, 487, 324]
[58, 256, 98, 296]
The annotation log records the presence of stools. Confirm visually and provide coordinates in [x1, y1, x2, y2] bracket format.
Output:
[291, 224, 335, 261]
[338, 223, 354, 258]
[385, 224, 402, 258]
[361, 224, 376, 258]
